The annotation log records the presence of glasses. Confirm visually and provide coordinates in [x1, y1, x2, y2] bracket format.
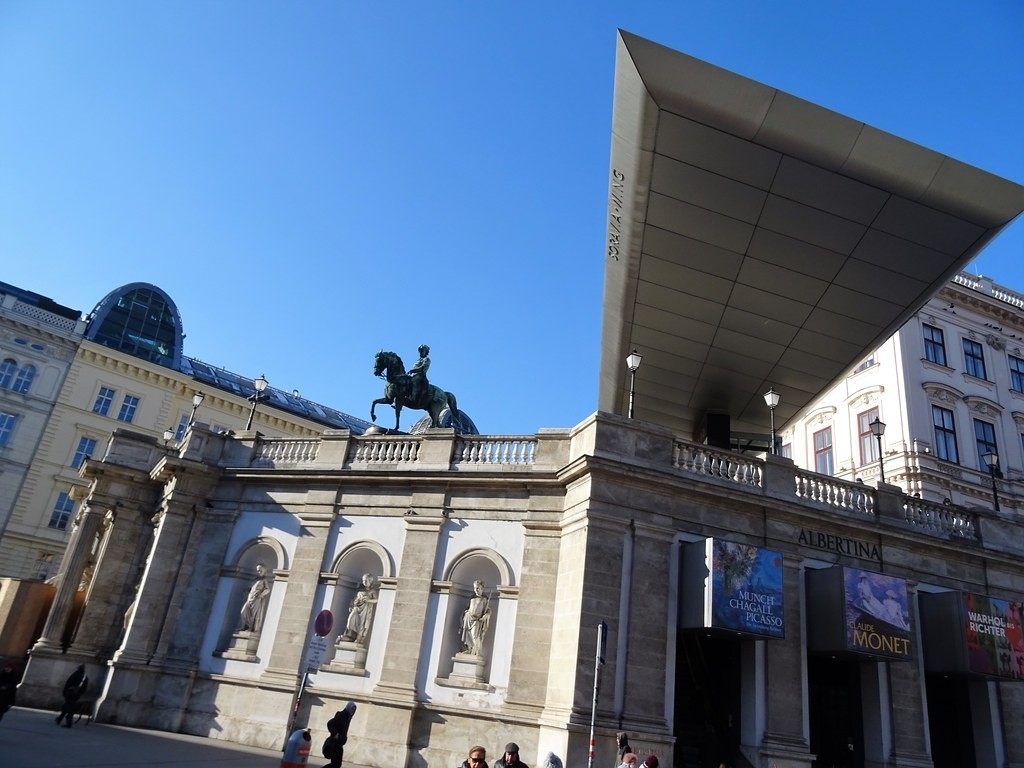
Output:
[470, 757, 484, 764]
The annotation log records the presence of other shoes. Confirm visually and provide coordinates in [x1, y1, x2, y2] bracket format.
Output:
[56, 717, 63, 724]
[63, 719, 72, 728]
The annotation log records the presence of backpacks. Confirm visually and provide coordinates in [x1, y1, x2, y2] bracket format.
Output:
[323, 734, 342, 759]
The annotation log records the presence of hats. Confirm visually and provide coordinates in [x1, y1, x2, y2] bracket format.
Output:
[646, 755, 658, 768]
[914, 493, 920, 496]
[856, 478, 862, 481]
[505, 742, 519, 751]
[946, 499, 950, 501]
[623, 753, 638, 764]
[858, 572, 868, 577]
[886, 590, 898, 598]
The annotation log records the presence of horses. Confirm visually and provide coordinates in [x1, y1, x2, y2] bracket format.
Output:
[370, 348, 461, 431]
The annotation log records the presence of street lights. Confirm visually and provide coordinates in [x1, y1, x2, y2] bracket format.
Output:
[982, 449, 1001, 512]
[763, 386, 781, 455]
[869, 416, 886, 482]
[627, 347, 643, 419]
[182, 390, 205, 437]
[246, 374, 267, 429]
[163, 427, 174, 446]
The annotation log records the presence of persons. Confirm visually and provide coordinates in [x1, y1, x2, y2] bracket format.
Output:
[55, 664, 89, 728]
[405, 344, 431, 410]
[618, 753, 639, 768]
[320, 703, 356, 768]
[614, 732, 632, 768]
[457, 746, 488, 768]
[0, 661, 17, 721]
[235, 563, 270, 632]
[639, 755, 659, 768]
[543, 752, 563, 768]
[341, 573, 377, 643]
[461, 579, 492, 657]
[494, 743, 529, 768]
